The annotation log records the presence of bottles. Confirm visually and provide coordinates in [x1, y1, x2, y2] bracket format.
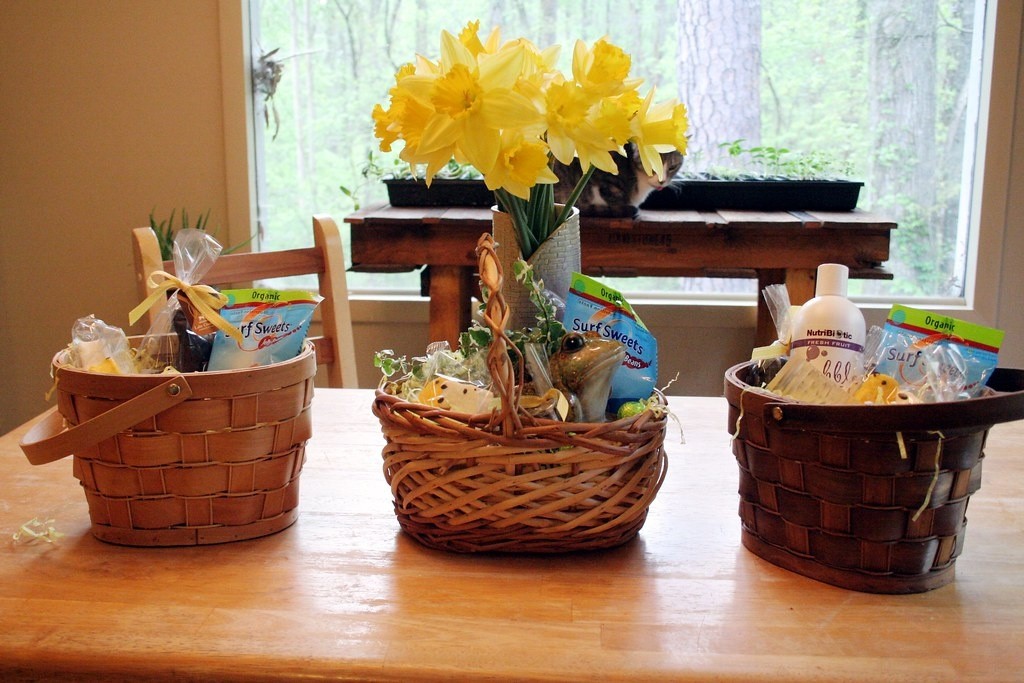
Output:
[790, 263, 866, 388]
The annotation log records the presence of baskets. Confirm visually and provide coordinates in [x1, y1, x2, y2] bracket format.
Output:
[18, 332, 314, 548]
[375, 374, 672, 553]
[725, 352, 1023, 596]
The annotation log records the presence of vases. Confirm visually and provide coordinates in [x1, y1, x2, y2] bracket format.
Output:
[485, 203, 582, 336]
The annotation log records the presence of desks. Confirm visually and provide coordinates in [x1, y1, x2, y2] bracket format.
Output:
[344, 201, 900, 351]
[0, 384, 1024, 683]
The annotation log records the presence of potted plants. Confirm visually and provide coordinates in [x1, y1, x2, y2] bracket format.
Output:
[338, 137, 864, 211]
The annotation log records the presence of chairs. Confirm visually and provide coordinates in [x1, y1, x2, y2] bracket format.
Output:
[135, 216, 361, 388]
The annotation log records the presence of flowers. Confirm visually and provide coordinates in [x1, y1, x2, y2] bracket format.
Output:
[371, 19, 692, 253]
